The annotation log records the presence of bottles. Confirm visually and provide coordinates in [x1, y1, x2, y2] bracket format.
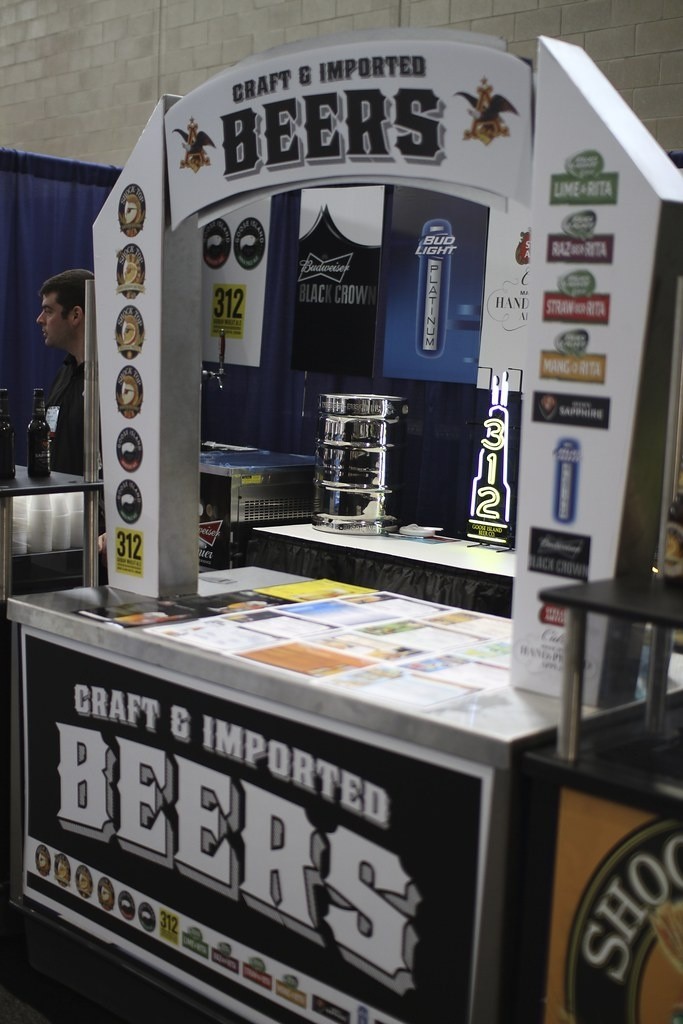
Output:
[27, 387, 51, 478]
[0, 389, 15, 480]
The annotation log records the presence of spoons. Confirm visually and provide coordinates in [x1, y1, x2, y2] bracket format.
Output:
[406, 524, 444, 531]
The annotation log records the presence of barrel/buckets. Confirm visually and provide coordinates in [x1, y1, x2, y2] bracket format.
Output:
[312, 393, 409, 534]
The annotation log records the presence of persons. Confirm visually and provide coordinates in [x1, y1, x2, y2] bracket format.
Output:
[37, 268, 107, 560]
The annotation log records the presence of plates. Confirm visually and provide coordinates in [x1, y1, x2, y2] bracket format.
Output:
[399, 527, 435, 537]
